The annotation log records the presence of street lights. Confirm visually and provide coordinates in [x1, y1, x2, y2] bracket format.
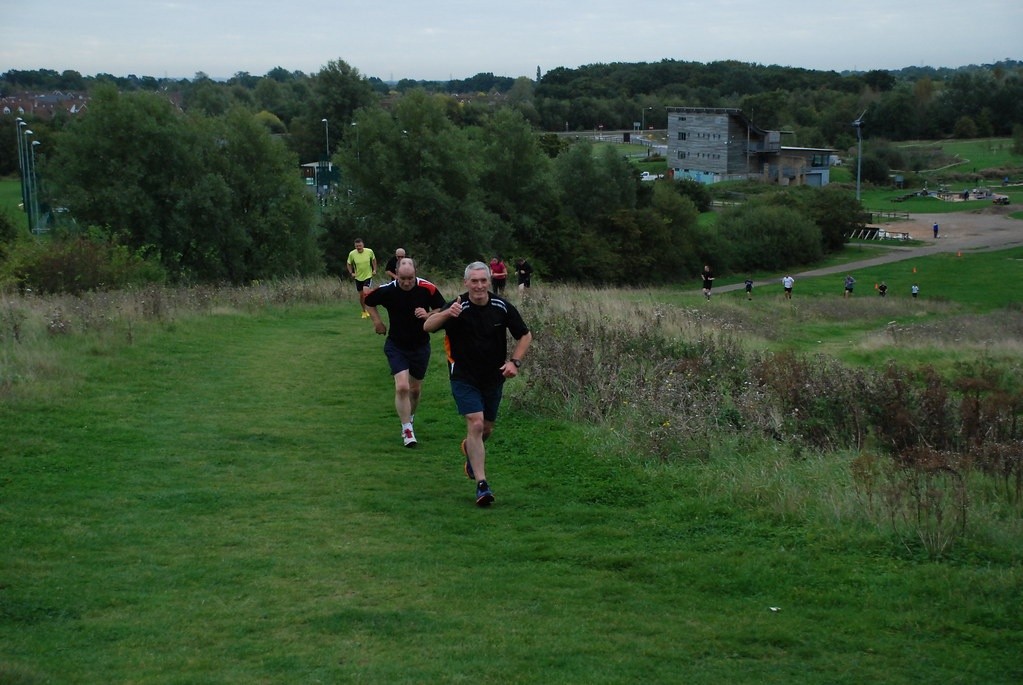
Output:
[15, 116, 41, 235]
[641, 106, 653, 130]
[322, 119, 330, 156]
[351, 123, 360, 165]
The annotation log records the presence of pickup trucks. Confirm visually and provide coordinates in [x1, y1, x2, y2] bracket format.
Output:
[639, 172, 664, 181]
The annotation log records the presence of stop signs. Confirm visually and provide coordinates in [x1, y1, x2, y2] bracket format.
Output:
[599, 124, 603, 129]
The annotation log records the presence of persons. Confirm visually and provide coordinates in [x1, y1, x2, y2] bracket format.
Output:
[911, 283, 919, 299]
[782, 273, 794, 300]
[490, 258, 508, 295]
[347, 238, 377, 319]
[514, 257, 534, 304]
[745, 277, 753, 301]
[844, 274, 856, 299]
[923, 185, 928, 196]
[423, 262, 533, 504]
[701, 265, 715, 301]
[363, 259, 447, 447]
[964, 187, 968, 201]
[933, 221, 938, 238]
[385, 248, 411, 281]
[879, 281, 888, 298]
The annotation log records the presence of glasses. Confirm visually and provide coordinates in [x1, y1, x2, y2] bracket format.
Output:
[397, 255, 404, 258]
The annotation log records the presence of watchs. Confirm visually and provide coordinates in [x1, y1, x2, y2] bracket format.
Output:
[510, 358, 521, 368]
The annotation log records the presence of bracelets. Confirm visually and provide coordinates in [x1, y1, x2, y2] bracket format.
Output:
[351, 272, 352, 274]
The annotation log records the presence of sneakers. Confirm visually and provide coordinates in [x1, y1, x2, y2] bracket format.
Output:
[475, 480, 494, 507]
[362, 311, 369, 318]
[462, 439, 476, 479]
[401, 428, 417, 447]
